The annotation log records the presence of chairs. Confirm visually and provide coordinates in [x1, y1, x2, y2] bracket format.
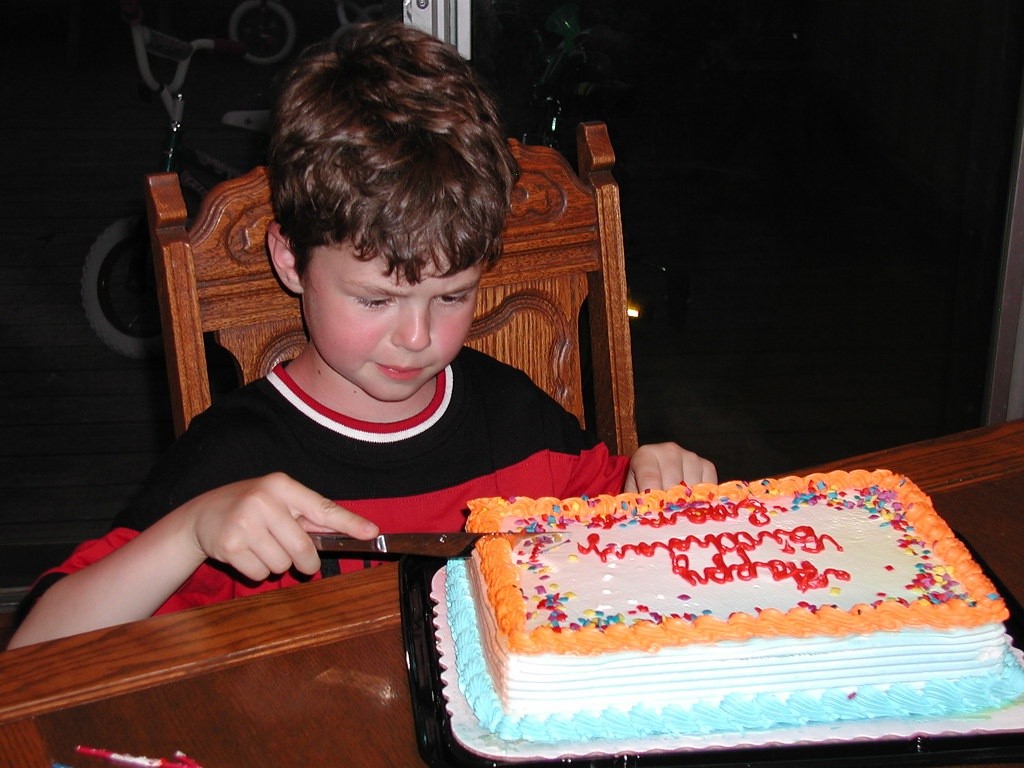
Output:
[141, 119, 639, 460]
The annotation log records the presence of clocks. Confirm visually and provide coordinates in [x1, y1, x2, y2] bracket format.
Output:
[82, 212, 165, 360]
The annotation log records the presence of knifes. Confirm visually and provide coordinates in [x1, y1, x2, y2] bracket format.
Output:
[299, 530, 574, 558]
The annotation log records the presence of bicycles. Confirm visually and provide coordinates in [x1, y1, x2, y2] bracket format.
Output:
[80, 0, 282, 364]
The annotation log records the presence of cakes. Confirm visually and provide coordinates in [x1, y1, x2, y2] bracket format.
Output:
[444, 467, 1024, 744]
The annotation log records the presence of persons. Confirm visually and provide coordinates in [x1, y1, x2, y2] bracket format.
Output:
[5, 19, 717, 650]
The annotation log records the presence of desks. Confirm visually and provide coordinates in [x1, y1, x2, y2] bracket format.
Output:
[0, 414, 1024, 768]
